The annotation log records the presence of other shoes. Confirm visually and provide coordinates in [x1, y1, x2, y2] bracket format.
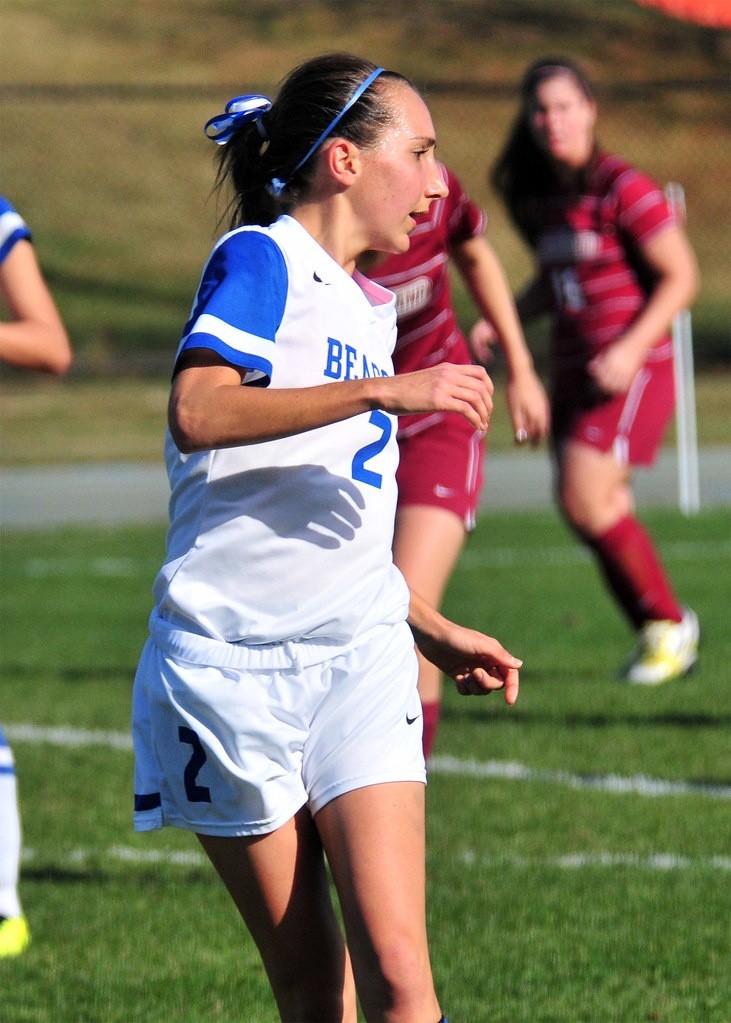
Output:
[0, 889, 28, 958]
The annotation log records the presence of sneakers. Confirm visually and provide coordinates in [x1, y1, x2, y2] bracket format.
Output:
[628, 608, 700, 686]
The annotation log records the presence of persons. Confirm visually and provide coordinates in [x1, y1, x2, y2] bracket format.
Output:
[0, 192, 73, 960]
[354, 161, 552, 761]
[132, 54, 523, 1023]
[469, 56, 701, 688]
